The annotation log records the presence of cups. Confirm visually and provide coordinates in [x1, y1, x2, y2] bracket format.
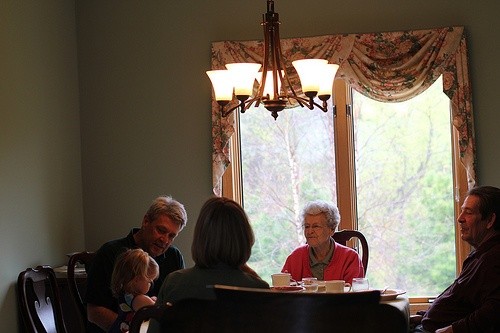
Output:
[325, 280, 351, 294]
[301, 277, 318, 293]
[271, 273, 292, 286]
[352, 278, 370, 293]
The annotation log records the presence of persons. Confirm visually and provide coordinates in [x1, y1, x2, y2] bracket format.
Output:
[85, 195, 187, 333]
[413, 185, 500, 333]
[155, 197, 270, 307]
[281, 205, 365, 287]
[111, 248, 159, 333]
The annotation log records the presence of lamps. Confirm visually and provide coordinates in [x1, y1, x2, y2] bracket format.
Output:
[206, 0, 340, 120]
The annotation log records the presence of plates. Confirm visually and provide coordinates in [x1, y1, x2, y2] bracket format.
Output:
[295, 281, 325, 288]
[372, 289, 407, 301]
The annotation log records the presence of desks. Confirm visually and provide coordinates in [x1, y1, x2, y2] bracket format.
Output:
[53, 264, 87, 279]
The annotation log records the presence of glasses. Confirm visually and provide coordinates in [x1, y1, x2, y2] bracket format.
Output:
[302, 223, 328, 230]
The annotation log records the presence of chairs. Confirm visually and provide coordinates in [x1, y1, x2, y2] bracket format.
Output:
[18, 231, 411, 333]
